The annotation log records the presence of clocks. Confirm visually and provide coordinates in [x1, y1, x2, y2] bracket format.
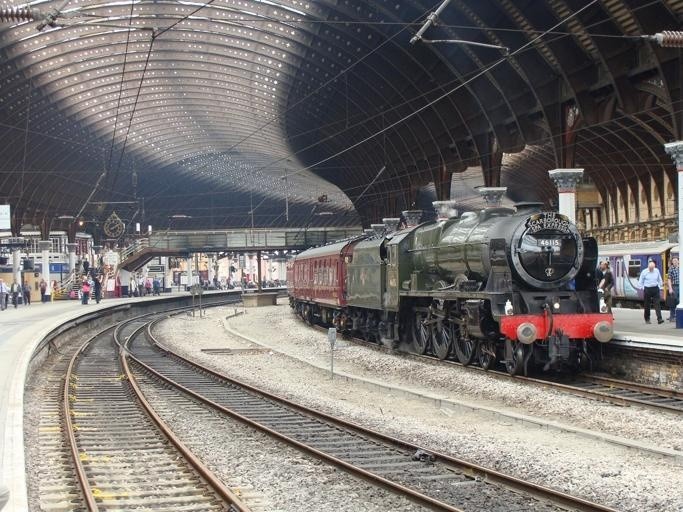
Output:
[103, 216, 123, 237]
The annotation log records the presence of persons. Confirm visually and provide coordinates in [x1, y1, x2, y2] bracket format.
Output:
[600, 260, 614, 313]
[666, 256, 680, 323]
[594, 261, 604, 300]
[81, 258, 90, 276]
[212, 272, 282, 291]
[0, 272, 161, 312]
[96, 253, 101, 271]
[638, 261, 665, 324]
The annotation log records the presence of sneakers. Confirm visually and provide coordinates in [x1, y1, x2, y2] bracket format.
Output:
[645, 321, 651, 324]
[658, 320, 664, 324]
